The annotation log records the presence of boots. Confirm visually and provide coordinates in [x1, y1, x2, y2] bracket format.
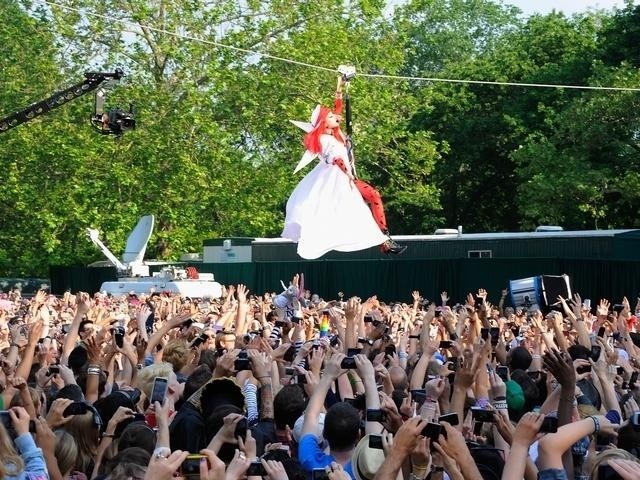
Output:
[380, 230, 408, 257]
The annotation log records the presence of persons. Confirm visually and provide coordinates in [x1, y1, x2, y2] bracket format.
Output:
[280, 75, 409, 261]
[1, 272, 639, 480]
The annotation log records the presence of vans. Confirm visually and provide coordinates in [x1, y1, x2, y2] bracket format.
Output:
[100, 268, 224, 299]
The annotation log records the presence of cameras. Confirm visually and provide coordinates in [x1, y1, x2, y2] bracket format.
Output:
[0, 300, 640, 480]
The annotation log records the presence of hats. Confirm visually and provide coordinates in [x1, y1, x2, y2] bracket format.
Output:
[351, 432, 385, 480]
[292, 413, 326, 445]
